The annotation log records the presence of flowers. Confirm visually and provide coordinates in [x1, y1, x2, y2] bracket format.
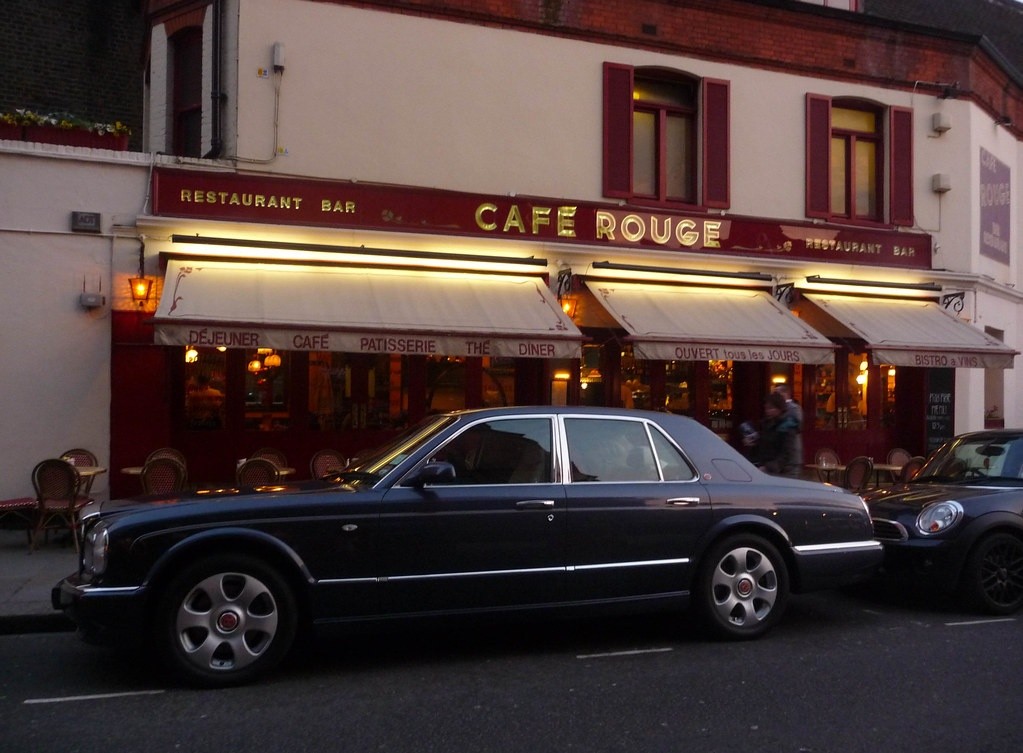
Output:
[0, 107, 132, 137]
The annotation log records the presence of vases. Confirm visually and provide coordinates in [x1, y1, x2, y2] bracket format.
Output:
[0, 121, 129, 151]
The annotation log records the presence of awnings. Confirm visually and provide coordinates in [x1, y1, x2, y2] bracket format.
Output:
[575, 273, 843, 372]
[143, 240, 585, 363]
[799, 289, 1022, 373]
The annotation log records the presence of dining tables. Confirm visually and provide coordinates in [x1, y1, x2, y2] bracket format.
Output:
[804, 463, 902, 488]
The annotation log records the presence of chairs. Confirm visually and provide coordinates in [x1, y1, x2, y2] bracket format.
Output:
[0, 448, 347, 554]
[500, 436, 553, 486]
[843, 456, 873, 490]
[901, 455, 927, 483]
[610, 444, 660, 480]
[886, 448, 911, 482]
[815, 448, 841, 482]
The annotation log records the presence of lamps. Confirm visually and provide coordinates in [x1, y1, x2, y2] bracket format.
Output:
[557, 268, 578, 320]
[943, 292, 972, 324]
[185, 346, 227, 362]
[248, 348, 281, 373]
[775, 282, 799, 318]
[129, 241, 153, 300]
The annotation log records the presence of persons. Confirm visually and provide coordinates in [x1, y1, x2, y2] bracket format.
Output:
[769, 383, 804, 480]
[738, 392, 801, 474]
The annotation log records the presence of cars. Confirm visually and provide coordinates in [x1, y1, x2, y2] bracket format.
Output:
[859, 429, 1022, 618]
[50, 402, 883, 691]
